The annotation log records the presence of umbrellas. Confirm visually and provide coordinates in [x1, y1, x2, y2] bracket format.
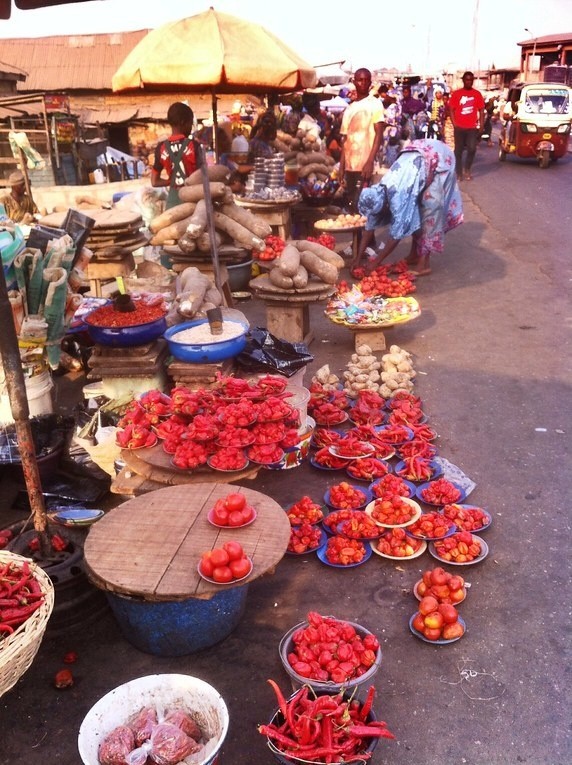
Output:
[113, 7, 317, 160]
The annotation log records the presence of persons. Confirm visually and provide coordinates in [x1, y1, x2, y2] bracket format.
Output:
[298, 95, 324, 147]
[249, 111, 277, 163]
[338, 68, 384, 254]
[151, 102, 204, 209]
[378, 77, 455, 151]
[449, 71, 485, 182]
[0, 171, 41, 225]
[350, 140, 463, 275]
[503, 94, 518, 146]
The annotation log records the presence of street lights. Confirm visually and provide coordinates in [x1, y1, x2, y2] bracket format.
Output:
[524, 28, 537, 79]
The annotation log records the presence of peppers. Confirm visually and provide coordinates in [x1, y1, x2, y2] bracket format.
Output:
[0, 560, 47, 641]
[307, 383, 424, 424]
[288, 612, 379, 683]
[257, 680, 395, 765]
[286, 474, 489, 562]
[313, 418, 436, 482]
[117, 371, 301, 471]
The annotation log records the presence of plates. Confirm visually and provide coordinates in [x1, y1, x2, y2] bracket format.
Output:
[231, 292, 251, 299]
[53, 509, 105, 526]
[112, 378, 491, 645]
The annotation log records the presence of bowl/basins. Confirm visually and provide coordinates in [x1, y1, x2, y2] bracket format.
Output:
[227, 258, 255, 289]
[164, 319, 249, 364]
[78, 674, 229, 765]
[9, 449, 62, 482]
[303, 196, 332, 207]
[81, 301, 168, 346]
[104, 585, 250, 658]
[245, 153, 285, 194]
[64, 296, 113, 347]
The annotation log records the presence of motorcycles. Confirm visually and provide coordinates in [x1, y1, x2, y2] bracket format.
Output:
[489, 83, 572, 169]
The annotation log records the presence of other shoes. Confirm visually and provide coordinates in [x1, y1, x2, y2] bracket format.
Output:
[407, 267, 432, 279]
[455, 172, 472, 182]
[401, 256, 421, 268]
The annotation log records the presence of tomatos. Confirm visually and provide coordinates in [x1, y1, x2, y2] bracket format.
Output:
[253, 235, 286, 260]
[333, 260, 418, 297]
[306, 235, 336, 250]
[199, 542, 250, 582]
[213, 493, 254, 526]
[411, 567, 466, 641]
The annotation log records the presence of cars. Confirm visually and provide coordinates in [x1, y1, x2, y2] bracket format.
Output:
[390, 82, 449, 111]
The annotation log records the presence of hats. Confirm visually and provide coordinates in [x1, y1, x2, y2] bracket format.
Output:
[3, 172, 26, 185]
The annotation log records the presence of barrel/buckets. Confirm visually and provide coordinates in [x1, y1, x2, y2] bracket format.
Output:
[277, 616, 382, 700]
[265, 687, 376, 765]
[0, 364, 53, 425]
[83, 381, 102, 399]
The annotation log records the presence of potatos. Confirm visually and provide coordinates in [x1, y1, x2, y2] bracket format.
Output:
[312, 344, 417, 399]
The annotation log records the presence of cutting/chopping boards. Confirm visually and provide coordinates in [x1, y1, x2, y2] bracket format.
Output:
[87, 339, 167, 379]
